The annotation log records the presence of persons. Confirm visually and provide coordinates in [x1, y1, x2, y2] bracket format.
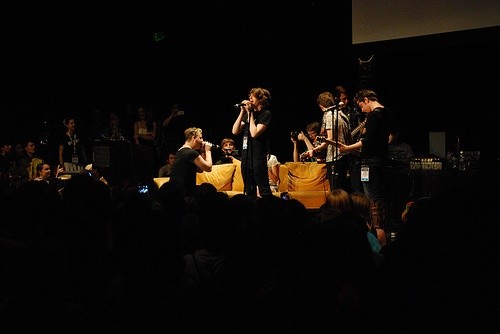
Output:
[169, 127, 213, 184]
[162, 103, 187, 153]
[0, 143, 12, 172]
[232, 88, 274, 196]
[0, 174, 451, 334]
[339, 89, 393, 228]
[34, 163, 50, 184]
[290, 122, 321, 162]
[59, 117, 83, 166]
[306, 92, 352, 191]
[216, 138, 241, 164]
[106, 118, 124, 139]
[134, 107, 157, 145]
[336, 85, 349, 109]
[18, 141, 43, 177]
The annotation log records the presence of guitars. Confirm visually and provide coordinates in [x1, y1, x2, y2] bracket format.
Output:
[316, 134, 362, 167]
[350, 118, 366, 138]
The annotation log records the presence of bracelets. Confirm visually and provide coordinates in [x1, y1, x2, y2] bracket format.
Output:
[201, 150, 205, 153]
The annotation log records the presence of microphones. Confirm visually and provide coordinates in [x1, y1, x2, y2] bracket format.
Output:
[323, 102, 344, 112]
[234, 104, 245, 107]
[212, 144, 220, 149]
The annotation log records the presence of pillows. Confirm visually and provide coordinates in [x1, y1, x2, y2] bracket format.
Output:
[229, 156, 245, 190]
[198, 163, 235, 191]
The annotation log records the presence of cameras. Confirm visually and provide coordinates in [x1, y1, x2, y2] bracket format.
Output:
[291, 131, 308, 140]
[281, 192, 289, 200]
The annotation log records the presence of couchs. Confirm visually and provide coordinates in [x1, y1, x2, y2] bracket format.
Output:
[154, 161, 330, 209]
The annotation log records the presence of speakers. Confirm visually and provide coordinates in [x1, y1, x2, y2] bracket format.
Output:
[92, 146, 113, 166]
